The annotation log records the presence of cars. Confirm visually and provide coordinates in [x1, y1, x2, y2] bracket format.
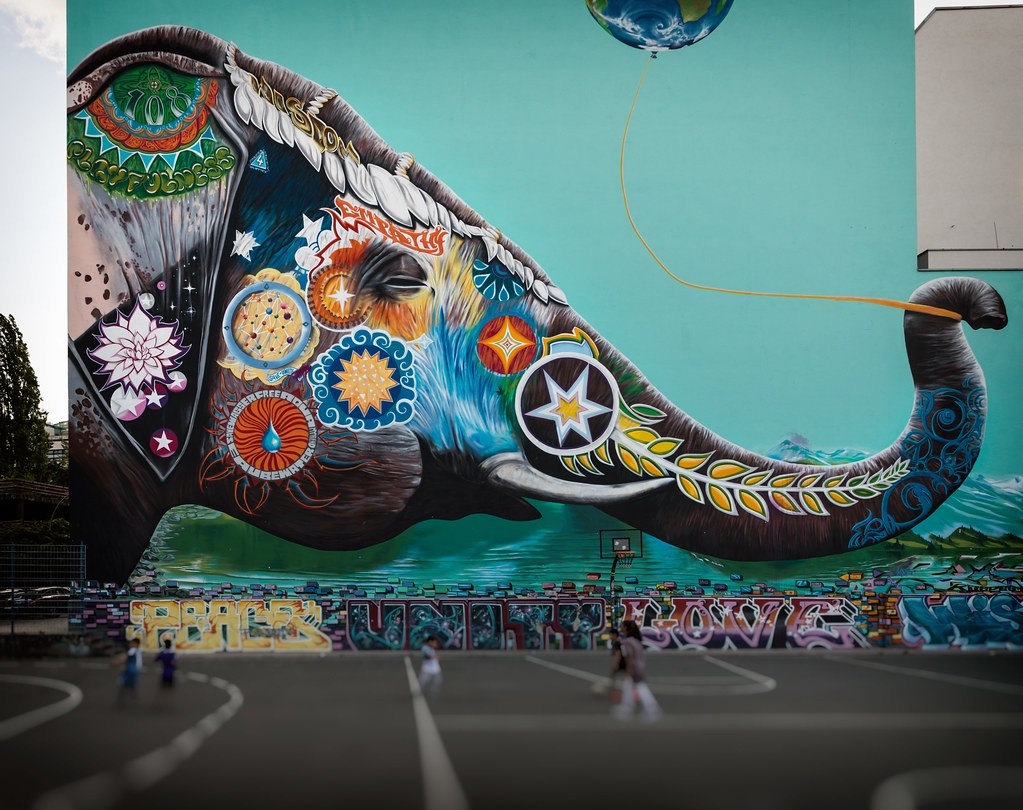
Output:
[0, 585, 72, 603]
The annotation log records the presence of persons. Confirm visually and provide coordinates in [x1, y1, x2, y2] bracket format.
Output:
[613, 619, 665, 722]
[608, 629, 644, 703]
[409, 635, 445, 705]
[153, 639, 180, 712]
[111, 637, 144, 712]
